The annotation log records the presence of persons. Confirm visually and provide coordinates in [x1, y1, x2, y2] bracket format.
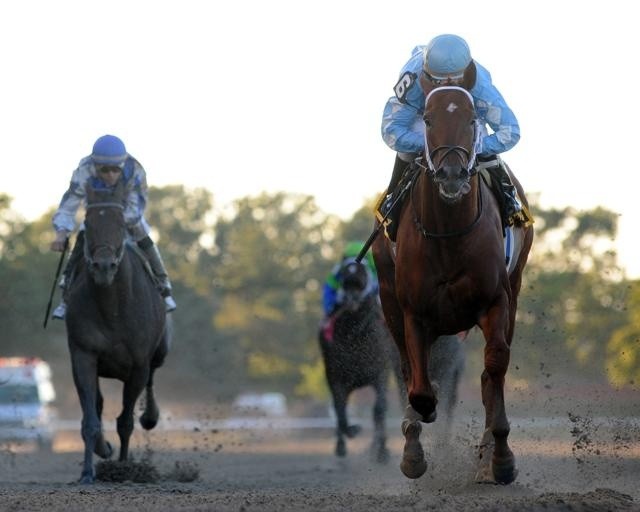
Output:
[379, 32, 520, 259]
[322, 242, 380, 340]
[53, 135, 178, 320]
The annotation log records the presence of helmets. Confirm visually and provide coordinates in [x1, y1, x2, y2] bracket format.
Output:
[424, 33, 471, 74]
[90, 134, 128, 164]
[341, 241, 370, 258]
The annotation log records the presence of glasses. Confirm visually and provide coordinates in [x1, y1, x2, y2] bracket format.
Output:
[422, 70, 465, 85]
[96, 167, 120, 174]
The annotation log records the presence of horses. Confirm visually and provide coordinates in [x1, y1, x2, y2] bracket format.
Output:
[60, 173, 168, 486]
[371, 59, 535, 486]
[394, 330, 466, 425]
[320, 256, 393, 467]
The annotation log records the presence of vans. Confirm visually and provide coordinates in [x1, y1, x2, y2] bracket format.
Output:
[0, 357, 61, 453]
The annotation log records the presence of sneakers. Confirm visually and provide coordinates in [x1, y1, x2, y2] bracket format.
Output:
[52, 301, 68, 320]
[163, 296, 177, 314]
